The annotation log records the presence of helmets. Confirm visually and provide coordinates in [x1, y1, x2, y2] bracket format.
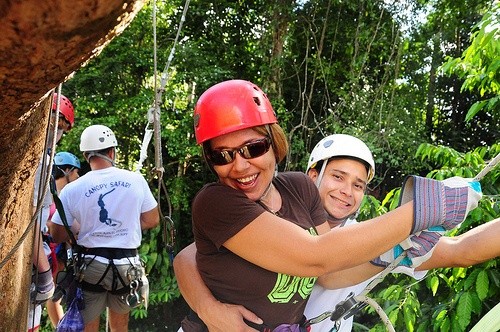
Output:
[194, 79, 279, 146]
[51, 93, 74, 128]
[80, 124, 119, 151]
[53, 151, 81, 170]
[305, 133, 375, 184]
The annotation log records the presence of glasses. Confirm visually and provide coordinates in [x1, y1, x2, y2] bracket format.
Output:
[206, 137, 272, 166]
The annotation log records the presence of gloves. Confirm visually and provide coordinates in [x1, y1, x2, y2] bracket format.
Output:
[399, 175, 483, 236]
[369, 232, 444, 267]
[30, 269, 56, 305]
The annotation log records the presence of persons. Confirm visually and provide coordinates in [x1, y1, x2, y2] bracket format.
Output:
[173, 134, 500, 332]
[48, 125, 159, 332]
[27, 92, 81, 332]
[178, 78, 482, 332]
